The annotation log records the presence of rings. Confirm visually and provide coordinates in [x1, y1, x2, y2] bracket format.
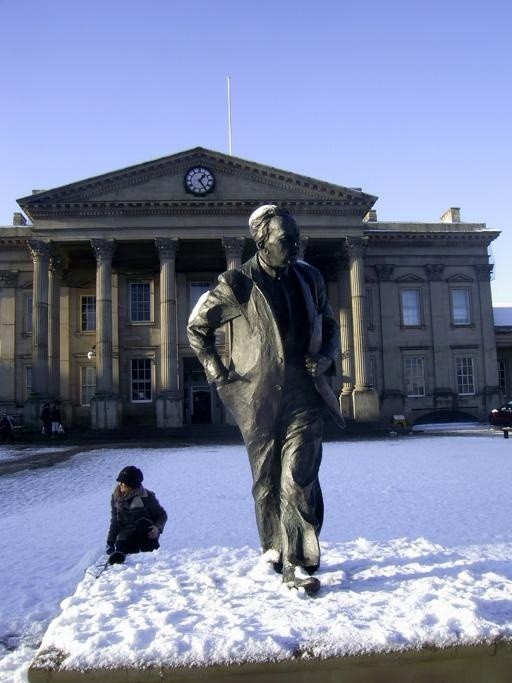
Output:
[152, 529, 154, 533]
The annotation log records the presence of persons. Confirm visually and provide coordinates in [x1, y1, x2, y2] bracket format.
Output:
[0, 408, 18, 443]
[40, 400, 55, 447]
[105, 465, 168, 555]
[186, 204, 345, 597]
[50, 402, 68, 442]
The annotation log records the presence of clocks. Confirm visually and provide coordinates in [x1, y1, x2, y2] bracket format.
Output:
[183, 165, 215, 197]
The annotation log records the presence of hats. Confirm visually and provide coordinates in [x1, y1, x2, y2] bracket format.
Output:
[116, 464, 143, 487]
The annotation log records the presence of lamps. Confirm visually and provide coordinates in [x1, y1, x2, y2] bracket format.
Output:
[88, 344, 96, 359]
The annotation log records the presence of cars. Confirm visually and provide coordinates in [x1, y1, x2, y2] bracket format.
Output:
[489, 404, 512, 425]
